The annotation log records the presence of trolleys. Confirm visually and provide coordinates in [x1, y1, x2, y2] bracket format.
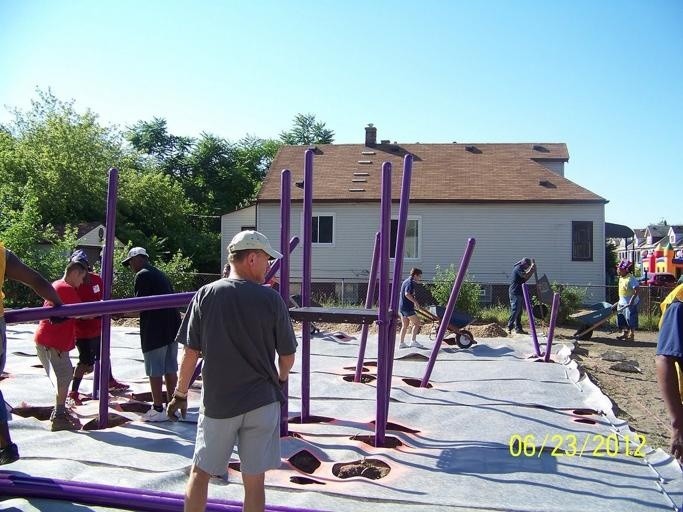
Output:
[532, 258, 555, 318]
[414, 304, 477, 348]
[569, 301, 631, 341]
[289, 294, 322, 335]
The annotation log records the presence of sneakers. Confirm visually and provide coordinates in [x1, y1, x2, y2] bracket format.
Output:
[410, 340, 423, 347]
[166, 409, 180, 417]
[625, 337, 634, 341]
[68, 392, 82, 405]
[0, 443, 19, 465]
[109, 380, 129, 389]
[143, 409, 166, 421]
[516, 329, 528, 334]
[619, 335, 629, 339]
[399, 343, 410, 348]
[52, 417, 75, 431]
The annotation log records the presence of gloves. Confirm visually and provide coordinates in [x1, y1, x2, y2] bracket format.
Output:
[167, 390, 188, 422]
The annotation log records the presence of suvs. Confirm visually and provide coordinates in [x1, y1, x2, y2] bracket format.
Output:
[639, 272, 676, 287]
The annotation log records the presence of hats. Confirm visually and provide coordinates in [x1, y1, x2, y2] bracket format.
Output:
[227, 229, 284, 260]
[122, 247, 149, 267]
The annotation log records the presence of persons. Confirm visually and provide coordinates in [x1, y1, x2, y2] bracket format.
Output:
[617, 261, 640, 341]
[122, 246, 181, 422]
[654, 277, 683, 462]
[507, 257, 535, 335]
[34, 262, 87, 432]
[66, 249, 130, 406]
[400, 268, 423, 350]
[167, 230, 298, 512]
[0, 242, 63, 465]
